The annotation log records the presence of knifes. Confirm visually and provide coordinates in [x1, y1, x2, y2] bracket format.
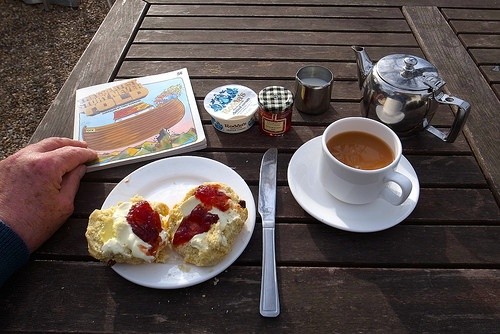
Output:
[258, 148, 280, 317]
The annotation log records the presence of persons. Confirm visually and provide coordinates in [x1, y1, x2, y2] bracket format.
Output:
[0, 136, 100, 286]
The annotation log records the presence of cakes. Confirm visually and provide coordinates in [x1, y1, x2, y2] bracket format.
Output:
[84, 181, 247, 268]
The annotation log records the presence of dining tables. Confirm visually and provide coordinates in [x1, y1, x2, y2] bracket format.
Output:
[0, 0, 500, 334]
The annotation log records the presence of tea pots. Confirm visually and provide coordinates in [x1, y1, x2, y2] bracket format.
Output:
[349, 44, 471, 143]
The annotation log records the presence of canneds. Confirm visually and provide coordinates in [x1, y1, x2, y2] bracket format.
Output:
[257, 86, 294, 137]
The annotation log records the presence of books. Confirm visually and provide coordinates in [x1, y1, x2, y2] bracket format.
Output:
[73, 67, 207, 173]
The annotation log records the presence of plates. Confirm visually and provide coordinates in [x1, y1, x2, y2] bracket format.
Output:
[100, 156, 256, 289]
[287, 134, 420, 232]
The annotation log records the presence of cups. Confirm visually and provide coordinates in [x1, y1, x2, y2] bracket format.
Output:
[295, 65, 333, 115]
[321, 117, 413, 206]
